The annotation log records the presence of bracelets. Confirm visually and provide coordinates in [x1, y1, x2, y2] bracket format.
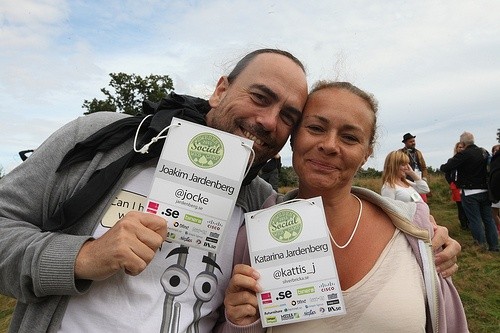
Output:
[422, 177, 427, 181]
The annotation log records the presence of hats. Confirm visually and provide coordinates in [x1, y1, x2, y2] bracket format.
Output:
[402, 133, 415, 142]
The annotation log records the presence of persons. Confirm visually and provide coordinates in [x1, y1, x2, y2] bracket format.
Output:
[441, 132, 500, 252]
[380, 150, 431, 203]
[0, 47, 462, 333]
[217, 81, 469, 333]
[400, 132, 427, 203]
[258, 153, 281, 194]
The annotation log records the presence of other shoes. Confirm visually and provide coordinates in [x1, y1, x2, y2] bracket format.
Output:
[467, 225, 472, 230]
[461, 226, 466, 230]
[489, 247, 500, 252]
[477, 245, 488, 252]
